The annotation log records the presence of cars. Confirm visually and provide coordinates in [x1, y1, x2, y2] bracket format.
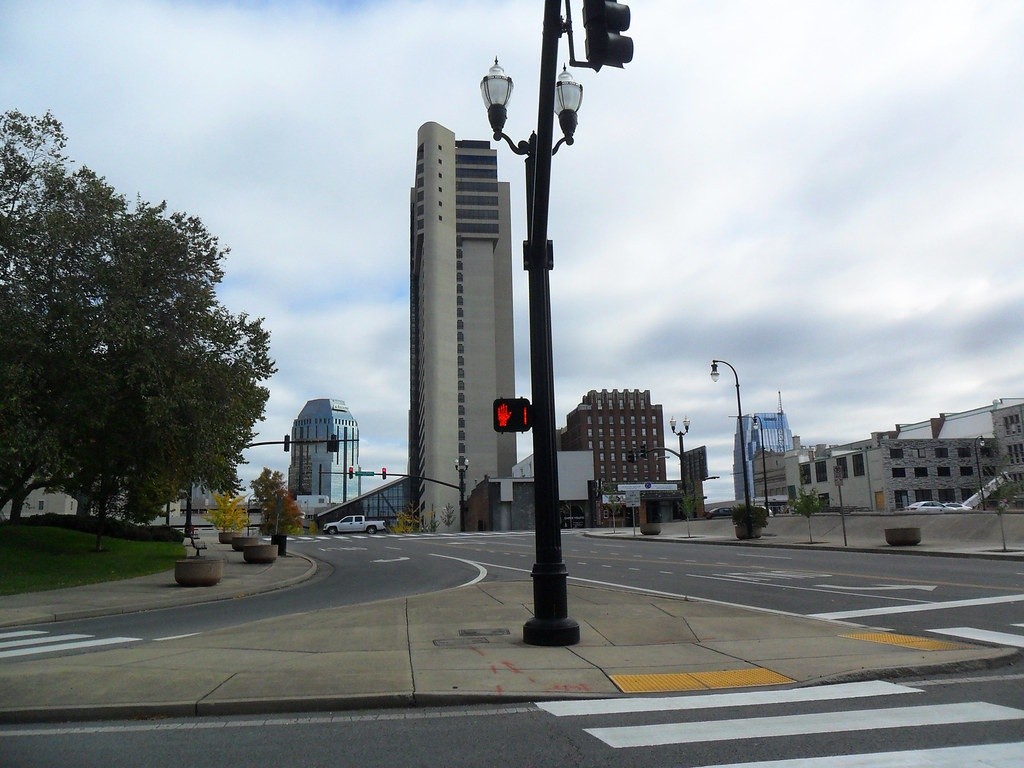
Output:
[708, 507, 733, 516]
[903, 500, 973, 512]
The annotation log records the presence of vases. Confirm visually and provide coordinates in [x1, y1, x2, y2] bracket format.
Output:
[231, 536, 259, 552]
[639, 523, 662, 535]
[242, 544, 278, 564]
[884, 527, 922, 547]
[174, 558, 226, 587]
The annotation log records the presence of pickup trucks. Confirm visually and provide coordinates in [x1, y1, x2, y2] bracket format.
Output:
[323, 514, 386, 535]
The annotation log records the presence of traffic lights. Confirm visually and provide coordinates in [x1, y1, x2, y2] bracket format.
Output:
[581, 0, 634, 71]
[640, 445, 646, 457]
[492, 398, 530, 432]
[382, 468, 386, 479]
[349, 467, 353, 479]
[628, 449, 634, 463]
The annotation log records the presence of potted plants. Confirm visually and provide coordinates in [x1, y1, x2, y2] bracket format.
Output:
[730, 498, 769, 540]
[250, 467, 305, 557]
[200, 487, 254, 544]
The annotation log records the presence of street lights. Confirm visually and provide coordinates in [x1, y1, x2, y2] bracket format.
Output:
[752, 416, 770, 516]
[454, 458, 469, 532]
[477, 53, 584, 648]
[710, 359, 754, 539]
[974, 435, 986, 512]
[670, 414, 691, 522]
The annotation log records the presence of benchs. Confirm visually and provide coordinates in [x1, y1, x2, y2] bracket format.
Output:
[185, 528, 200, 546]
[189, 535, 208, 559]
